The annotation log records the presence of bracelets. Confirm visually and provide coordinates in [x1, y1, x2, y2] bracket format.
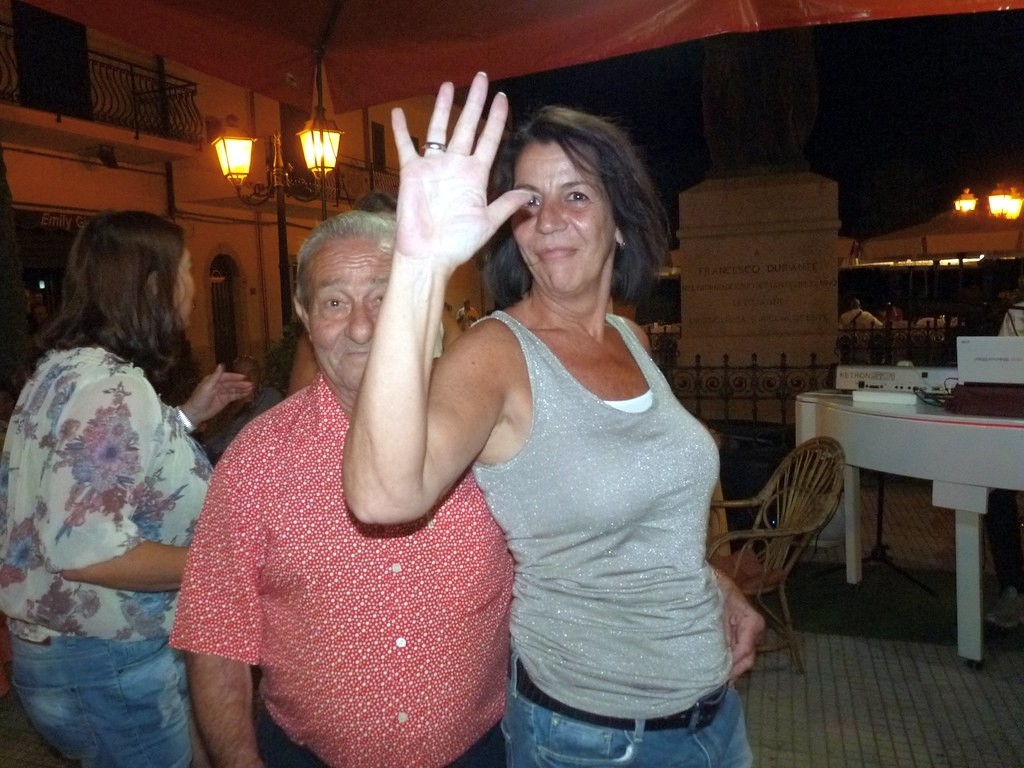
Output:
[178, 411, 197, 432]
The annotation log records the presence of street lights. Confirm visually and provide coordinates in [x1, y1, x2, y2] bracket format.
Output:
[210, 104, 344, 387]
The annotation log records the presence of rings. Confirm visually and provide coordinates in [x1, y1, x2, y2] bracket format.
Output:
[422, 143, 446, 150]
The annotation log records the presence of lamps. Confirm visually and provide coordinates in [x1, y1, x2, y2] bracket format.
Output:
[96, 144, 119, 169]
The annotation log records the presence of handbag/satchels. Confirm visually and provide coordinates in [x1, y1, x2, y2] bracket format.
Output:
[913, 381, 1024, 417]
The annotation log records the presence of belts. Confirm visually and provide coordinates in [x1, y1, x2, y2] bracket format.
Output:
[506, 656, 728, 732]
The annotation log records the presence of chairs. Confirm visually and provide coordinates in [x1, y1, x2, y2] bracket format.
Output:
[706, 434, 846, 675]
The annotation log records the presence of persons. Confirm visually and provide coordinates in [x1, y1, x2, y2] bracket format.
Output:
[23, 286, 49, 333]
[341, 73, 764, 768]
[984, 277, 1024, 630]
[173, 211, 513, 768]
[0, 210, 255, 767]
[206, 356, 280, 457]
[841, 299, 881, 328]
[457, 298, 478, 328]
[287, 189, 462, 397]
